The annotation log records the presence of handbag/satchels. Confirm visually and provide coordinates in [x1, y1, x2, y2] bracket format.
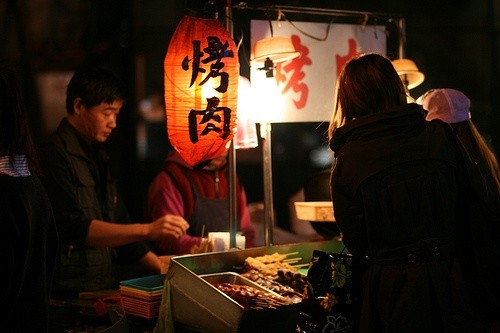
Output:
[295, 246, 353, 332]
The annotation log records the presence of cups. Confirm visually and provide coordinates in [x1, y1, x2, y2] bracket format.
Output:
[191, 248, 212, 254]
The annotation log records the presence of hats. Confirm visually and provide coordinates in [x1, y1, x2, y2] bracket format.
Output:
[415, 88, 471, 125]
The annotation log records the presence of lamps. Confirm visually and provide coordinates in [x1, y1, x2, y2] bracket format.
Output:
[391, 19, 424, 92]
[248, 9, 301, 63]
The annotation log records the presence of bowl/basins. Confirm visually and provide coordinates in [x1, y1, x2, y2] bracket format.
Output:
[294, 202, 335, 223]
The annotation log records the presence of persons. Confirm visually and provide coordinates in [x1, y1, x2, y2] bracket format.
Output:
[416, 86, 500, 333]
[33, 65, 189, 333]
[306, 174, 339, 237]
[240, 163, 323, 244]
[146, 139, 263, 256]
[329, 54, 487, 333]
[0, 67, 59, 333]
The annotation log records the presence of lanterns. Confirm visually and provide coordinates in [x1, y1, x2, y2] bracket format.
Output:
[164, 14, 239, 169]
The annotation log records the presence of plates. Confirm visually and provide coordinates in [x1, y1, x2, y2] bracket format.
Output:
[119, 273, 168, 301]
